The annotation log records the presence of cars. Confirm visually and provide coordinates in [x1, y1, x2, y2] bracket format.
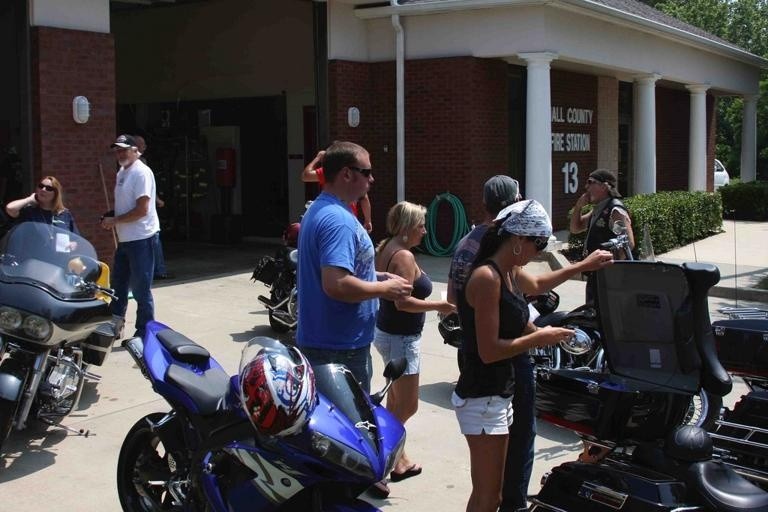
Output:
[713, 159, 730, 191]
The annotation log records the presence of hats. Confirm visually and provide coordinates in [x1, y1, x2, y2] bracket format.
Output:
[588, 169, 622, 198]
[110, 134, 136, 148]
[482, 174, 520, 208]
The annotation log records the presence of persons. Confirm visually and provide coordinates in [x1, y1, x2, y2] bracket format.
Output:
[570, 169, 638, 304]
[133, 136, 176, 280]
[447, 175, 614, 512]
[450, 199, 576, 512]
[98, 135, 161, 346]
[6, 176, 82, 266]
[372, 201, 457, 481]
[294, 141, 414, 395]
[302, 140, 373, 234]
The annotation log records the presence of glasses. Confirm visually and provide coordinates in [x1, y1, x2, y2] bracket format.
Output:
[350, 167, 370, 177]
[530, 236, 548, 250]
[588, 179, 602, 185]
[38, 182, 53, 191]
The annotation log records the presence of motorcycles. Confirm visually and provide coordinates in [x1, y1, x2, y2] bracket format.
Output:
[436, 259, 767, 512]
[0, 221, 124, 448]
[115, 321, 407, 512]
[251, 200, 313, 335]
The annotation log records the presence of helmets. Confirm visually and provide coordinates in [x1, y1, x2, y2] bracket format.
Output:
[282, 223, 300, 248]
[237, 336, 316, 439]
[436, 313, 466, 347]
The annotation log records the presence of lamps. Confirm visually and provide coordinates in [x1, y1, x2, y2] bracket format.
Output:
[347, 106, 360, 127]
[72, 95, 90, 125]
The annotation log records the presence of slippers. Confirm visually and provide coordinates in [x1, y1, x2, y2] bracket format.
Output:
[391, 463, 422, 482]
